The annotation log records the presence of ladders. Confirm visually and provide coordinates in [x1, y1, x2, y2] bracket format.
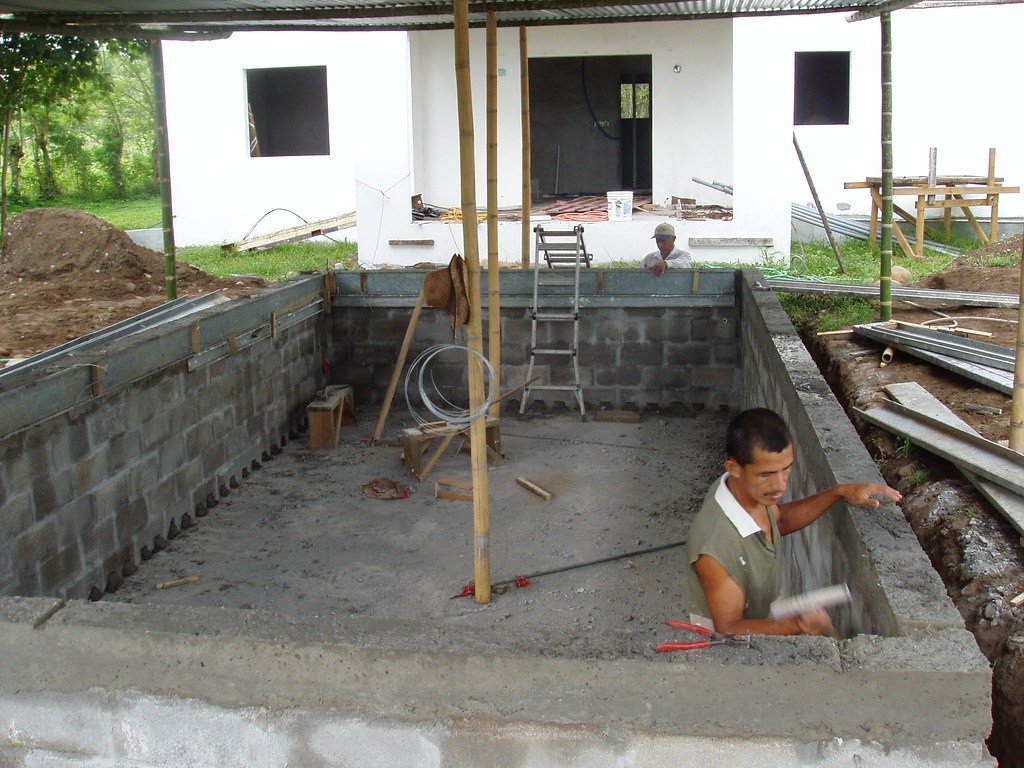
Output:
[518, 224, 593, 424]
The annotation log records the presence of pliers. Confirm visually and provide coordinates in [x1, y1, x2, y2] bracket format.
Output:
[654, 620, 751, 652]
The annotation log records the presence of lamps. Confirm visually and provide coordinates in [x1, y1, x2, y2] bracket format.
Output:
[674, 64, 681, 73]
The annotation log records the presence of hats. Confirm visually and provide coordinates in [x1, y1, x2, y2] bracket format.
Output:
[652, 222, 675, 240]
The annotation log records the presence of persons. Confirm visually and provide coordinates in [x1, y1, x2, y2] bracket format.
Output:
[687, 407, 903, 635]
[639, 222, 691, 278]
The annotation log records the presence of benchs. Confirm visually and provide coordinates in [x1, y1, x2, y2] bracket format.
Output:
[305, 384, 356, 450]
[401, 414, 500, 476]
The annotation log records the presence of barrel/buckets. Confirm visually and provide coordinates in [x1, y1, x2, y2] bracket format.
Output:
[606, 191, 633, 221]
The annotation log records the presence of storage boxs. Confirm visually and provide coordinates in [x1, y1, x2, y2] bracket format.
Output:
[531, 177, 544, 204]
